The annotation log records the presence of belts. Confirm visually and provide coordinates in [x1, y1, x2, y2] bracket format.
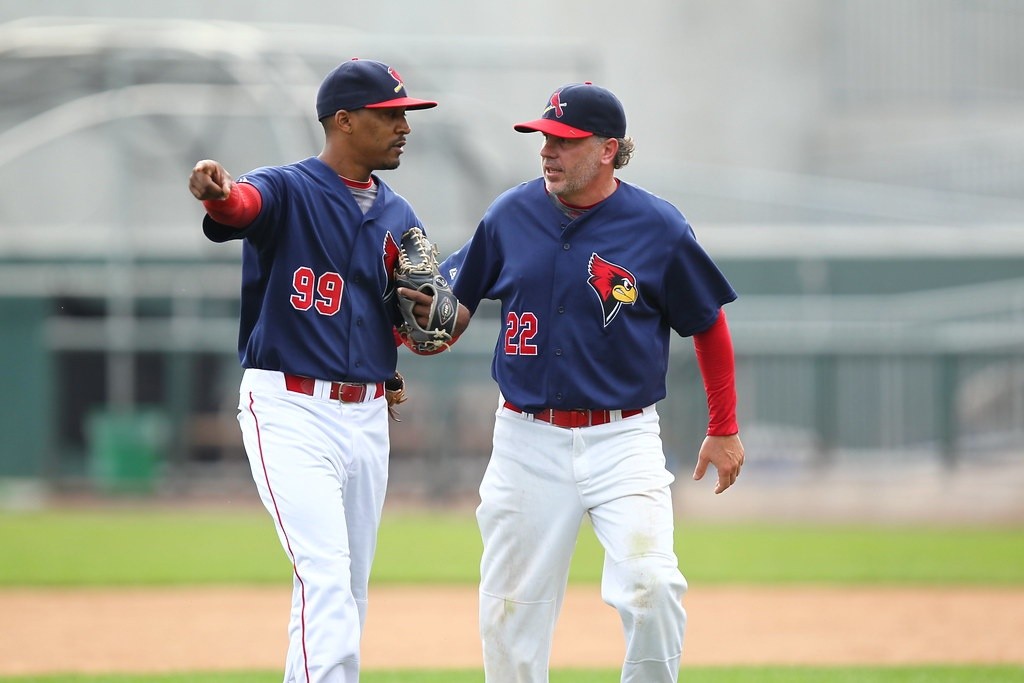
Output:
[499, 400, 644, 429]
[284, 374, 386, 405]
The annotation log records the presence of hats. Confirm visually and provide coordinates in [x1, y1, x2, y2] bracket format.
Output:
[316, 57, 438, 120]
[514, 81, 627, 139]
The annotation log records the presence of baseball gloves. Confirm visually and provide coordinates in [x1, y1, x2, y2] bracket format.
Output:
[395, 227, 459, 351]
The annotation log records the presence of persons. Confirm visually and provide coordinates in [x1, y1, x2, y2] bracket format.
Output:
[393, 81, 746, 683]
[190, 58, 470, 683]
